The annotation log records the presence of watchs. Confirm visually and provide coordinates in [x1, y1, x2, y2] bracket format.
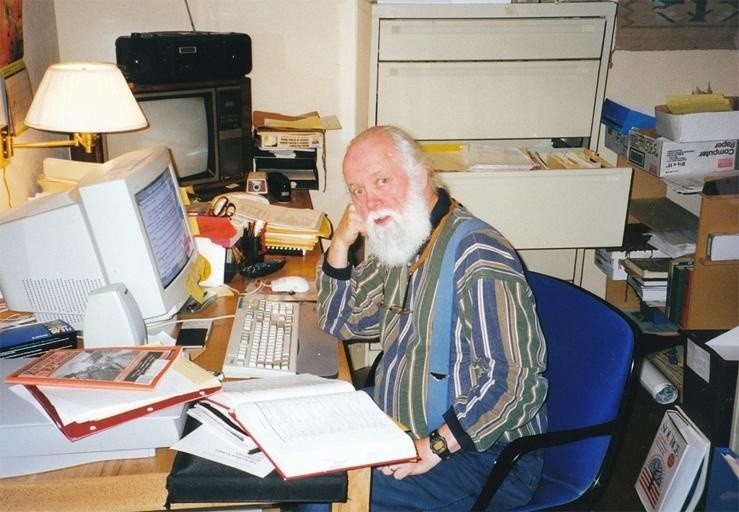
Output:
[427, 428, 452, 461]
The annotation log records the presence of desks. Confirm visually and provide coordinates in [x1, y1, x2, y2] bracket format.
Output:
[0, 190, 371, 512]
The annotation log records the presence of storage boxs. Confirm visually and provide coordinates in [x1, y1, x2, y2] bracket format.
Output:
[627, 129, 738, 178]
[681, 328, 739, 446]
[604, 126, 631, 156]
[600, 98, 657, 135]
[655, 96, 739, 142]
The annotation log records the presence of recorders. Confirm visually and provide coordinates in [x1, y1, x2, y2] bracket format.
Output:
[115, 31, 252, 83]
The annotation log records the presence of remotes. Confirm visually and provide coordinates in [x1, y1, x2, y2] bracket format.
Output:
[240, 256, 287, 278]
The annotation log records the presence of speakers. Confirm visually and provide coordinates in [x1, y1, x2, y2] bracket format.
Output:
[83, 283, 147, 350]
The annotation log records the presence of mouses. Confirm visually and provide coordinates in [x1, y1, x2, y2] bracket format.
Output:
[272, 276, 309, 293]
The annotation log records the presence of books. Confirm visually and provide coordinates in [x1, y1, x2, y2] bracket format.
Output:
[188, 201, 334, 257]
[633, 404, 712, 512]
[621, 256, 695, 330]
[3, 347, 222, 444]
[163, 364, 422, 512]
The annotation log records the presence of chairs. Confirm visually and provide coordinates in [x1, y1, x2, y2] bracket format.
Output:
[366, 272, 643, 512]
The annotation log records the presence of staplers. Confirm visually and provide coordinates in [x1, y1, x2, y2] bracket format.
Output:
[239, 254, 264, 271]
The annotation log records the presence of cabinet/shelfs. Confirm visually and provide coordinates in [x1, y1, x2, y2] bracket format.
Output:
[606, 157, 739, 336]
[358, 0, 632, 297]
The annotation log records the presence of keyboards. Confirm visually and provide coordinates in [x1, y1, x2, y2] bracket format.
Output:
[222, 291, 300, 379]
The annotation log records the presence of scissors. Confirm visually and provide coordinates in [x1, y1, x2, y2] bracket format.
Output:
[212, 196, 236, 218]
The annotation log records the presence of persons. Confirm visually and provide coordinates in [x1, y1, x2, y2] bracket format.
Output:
[293, 123, 551, 511]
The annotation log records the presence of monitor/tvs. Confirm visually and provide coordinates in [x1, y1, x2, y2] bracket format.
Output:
[95, 76, 256, 196]
[0, 147, 201, 341]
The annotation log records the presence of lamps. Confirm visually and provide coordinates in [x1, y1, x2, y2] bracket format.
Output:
[2, 64, 150, 168]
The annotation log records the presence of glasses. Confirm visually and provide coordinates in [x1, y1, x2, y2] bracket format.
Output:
[376, 267, 415, 314]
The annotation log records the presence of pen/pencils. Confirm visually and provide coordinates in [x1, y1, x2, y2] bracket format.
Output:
[244, 220, 267, 238]
